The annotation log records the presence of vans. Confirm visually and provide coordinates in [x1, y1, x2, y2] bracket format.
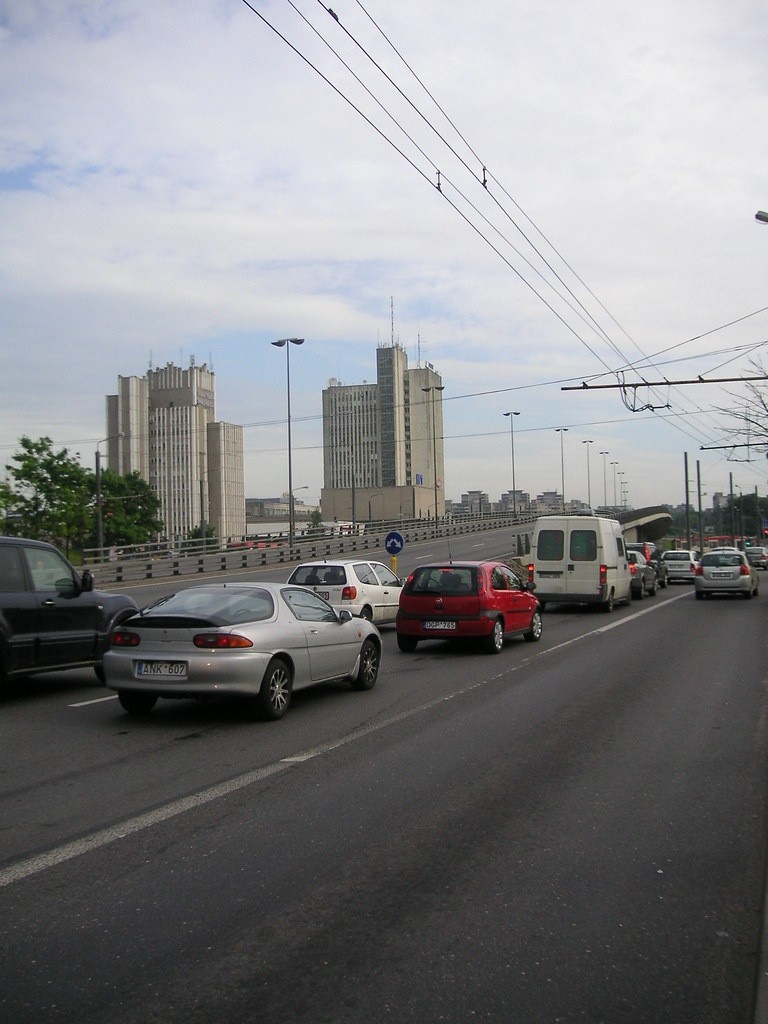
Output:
[527, 514, 631, 612]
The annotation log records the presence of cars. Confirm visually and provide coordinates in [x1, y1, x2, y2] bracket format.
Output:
[395, 560, 544, 655]
[102, 581, 384, 721]
[626, 549, 659, 599]
[287, 559, 407, 626]
[661, 544, 768, 598]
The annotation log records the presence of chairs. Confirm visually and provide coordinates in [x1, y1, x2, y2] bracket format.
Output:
[304, 575, 320, 584]
[439, 573, 456, 591]
[325, 572, 338, 582]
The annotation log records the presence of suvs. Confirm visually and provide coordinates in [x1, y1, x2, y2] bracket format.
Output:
[1, 536, 141, 696]
[624, 542, 670, 588]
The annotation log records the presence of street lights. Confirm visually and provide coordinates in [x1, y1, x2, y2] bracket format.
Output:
[503, 411, 522, 518]
[289, 485, 309, 537]
[420, 385, 446, 531]
[367, 491, 383, 523]
[399, 499, 413, 520]
[271, 337, 305, 558]
[581, 439, 595, 509]
[94, 431, 126, 562]
[599, 450, 628, 510]
[555, 427, 570, 511]
